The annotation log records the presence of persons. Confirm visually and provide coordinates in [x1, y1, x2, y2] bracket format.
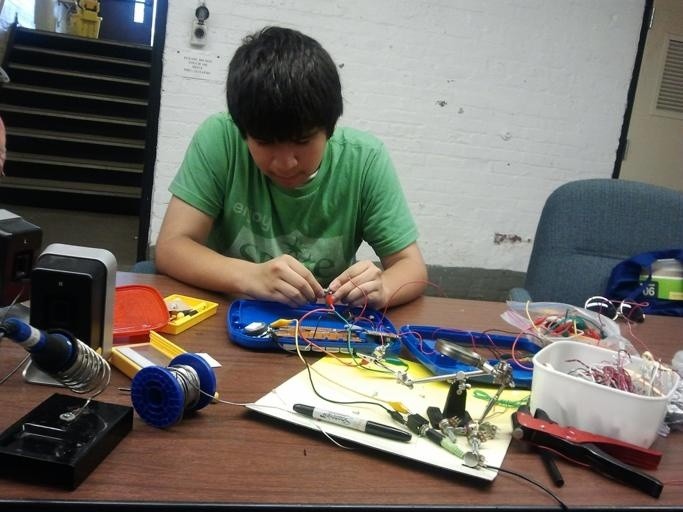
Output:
[154, 26, 430, 310]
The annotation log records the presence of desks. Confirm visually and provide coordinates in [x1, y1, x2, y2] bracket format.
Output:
[1, 266, 683, 512]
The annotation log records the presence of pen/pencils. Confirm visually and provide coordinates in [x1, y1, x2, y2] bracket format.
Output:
[294, 403, 412, 444]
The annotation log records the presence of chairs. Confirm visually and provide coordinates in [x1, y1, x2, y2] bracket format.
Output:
[509, 177, 682, 317]
